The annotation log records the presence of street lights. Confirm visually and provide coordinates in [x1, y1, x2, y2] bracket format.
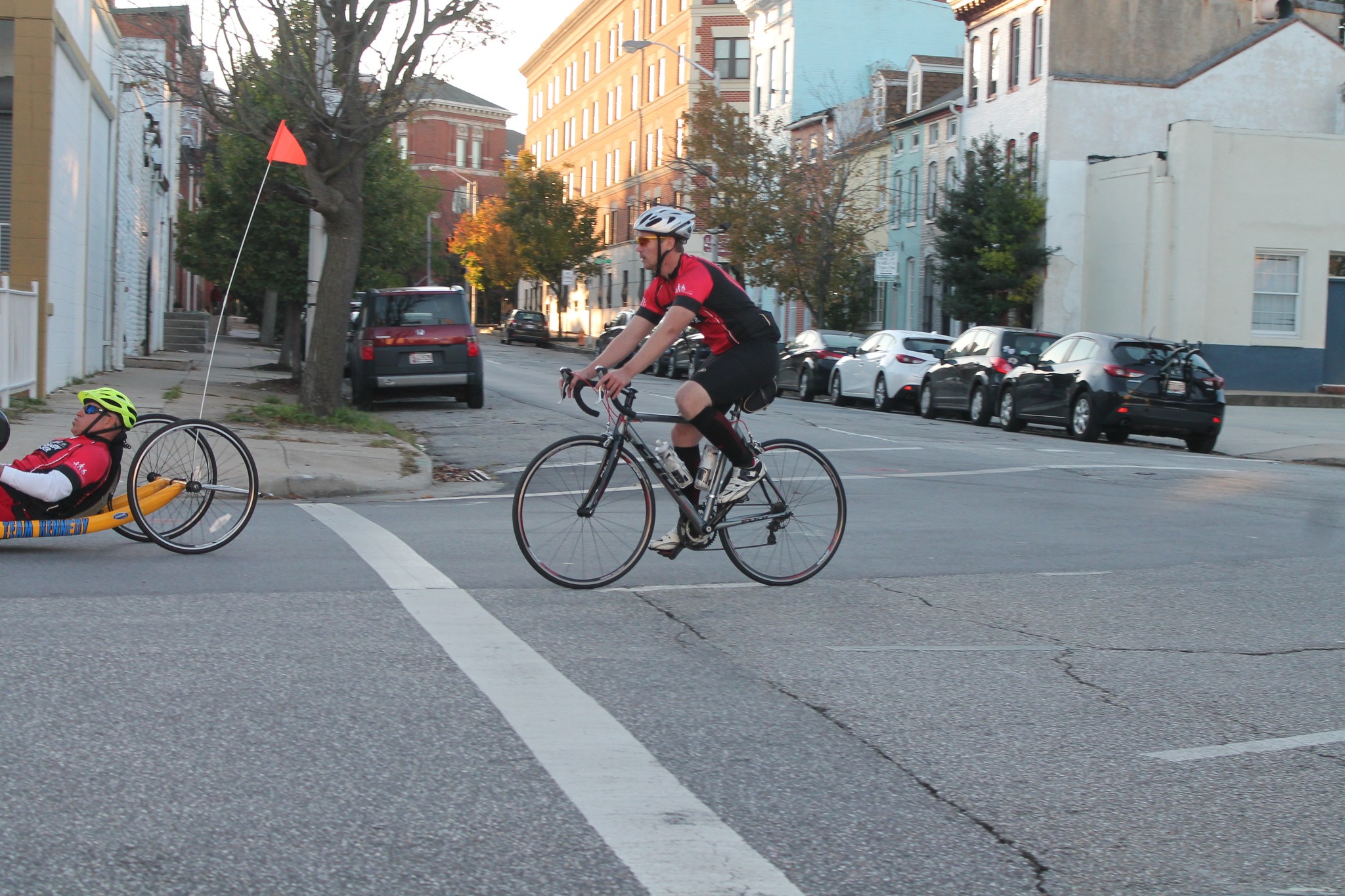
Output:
[622, 39, 721, 265]
[429, 164, 478, 326]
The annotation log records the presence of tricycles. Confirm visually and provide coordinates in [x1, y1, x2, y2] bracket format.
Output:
[0, 410, 258, 555]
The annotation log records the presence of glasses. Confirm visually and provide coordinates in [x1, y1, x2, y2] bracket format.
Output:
[83, 405, 117, 428]
[636, 236, 669, 247]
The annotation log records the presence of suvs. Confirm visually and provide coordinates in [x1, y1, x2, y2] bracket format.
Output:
[595, 308, 710, 379]
[300, 285, 484, 409]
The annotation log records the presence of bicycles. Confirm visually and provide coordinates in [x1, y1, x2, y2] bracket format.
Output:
[513, 366, 848, 589]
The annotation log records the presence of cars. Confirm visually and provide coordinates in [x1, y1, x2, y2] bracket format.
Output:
[829, 330, 956, 411]
[995, 331, 1227, 453]
[773, 331, 872, 401]
[917, 326, 1064, 425]
[501, 309, 550, 348]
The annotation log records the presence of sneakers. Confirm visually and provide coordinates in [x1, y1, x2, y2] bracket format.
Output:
[647, 522, 707, 551]
[717, 456, 767, 504]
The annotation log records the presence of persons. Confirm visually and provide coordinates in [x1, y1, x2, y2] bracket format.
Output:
[558, 207, 782, 551]
[0, 387, 137, 521]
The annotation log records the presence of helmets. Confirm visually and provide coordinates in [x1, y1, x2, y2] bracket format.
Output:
[633, 206, 696, 240]
[78, 387, 138, 428]
